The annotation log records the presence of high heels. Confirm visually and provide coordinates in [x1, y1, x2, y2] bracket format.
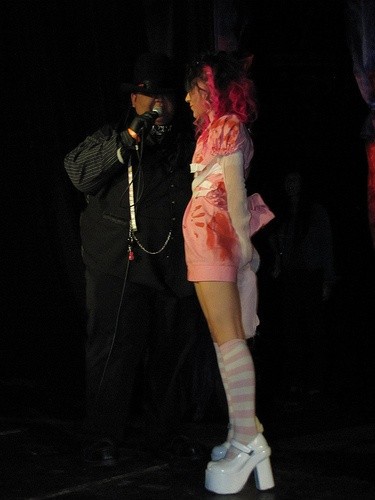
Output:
[204, 433, 275, 495]
[211, 442, 232, 461]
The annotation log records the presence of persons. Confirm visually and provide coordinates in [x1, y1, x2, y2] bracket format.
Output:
[62, 71, 200, 466]
[181, 51, 275, 495]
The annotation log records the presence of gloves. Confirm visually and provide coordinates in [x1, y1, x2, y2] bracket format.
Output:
[130, 111, 159, 139]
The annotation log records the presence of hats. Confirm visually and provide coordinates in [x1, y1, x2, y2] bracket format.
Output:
[120, 50, 186, 95]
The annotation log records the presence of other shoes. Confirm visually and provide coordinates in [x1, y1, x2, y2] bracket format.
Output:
[79, 433, 119, 463]
[287, 385, 305, 412]
[309, 385, 322, 396]
[138, 432, 210, 460]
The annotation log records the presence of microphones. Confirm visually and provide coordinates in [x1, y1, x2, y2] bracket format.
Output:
[139, 106, 162, 133]
